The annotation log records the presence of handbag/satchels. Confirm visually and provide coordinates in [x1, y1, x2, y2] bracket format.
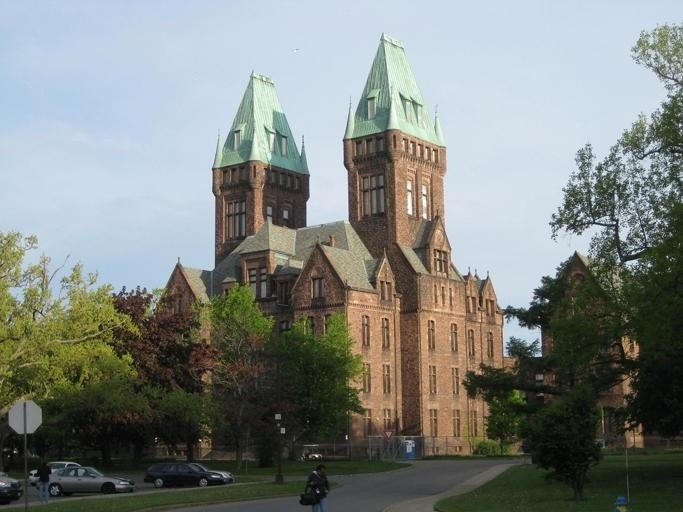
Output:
[300, 485, 321, 505]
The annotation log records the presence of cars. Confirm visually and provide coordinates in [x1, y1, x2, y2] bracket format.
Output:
[0, 474, 23, 505]
[28, 461, 135, 497]
[142, 460, 233, 488]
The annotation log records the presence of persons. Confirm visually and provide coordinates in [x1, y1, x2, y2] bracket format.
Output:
[307, 464, 330, 512]
[38, 461, 52, 504]
